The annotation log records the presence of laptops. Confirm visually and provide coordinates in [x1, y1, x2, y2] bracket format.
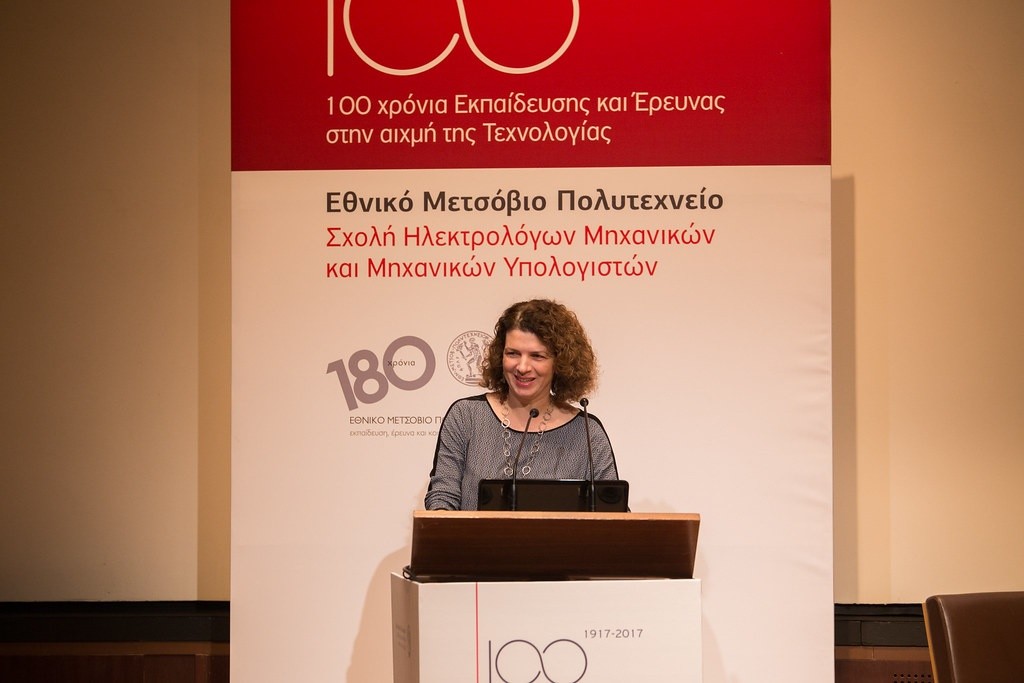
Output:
[478, 479, 629, 513]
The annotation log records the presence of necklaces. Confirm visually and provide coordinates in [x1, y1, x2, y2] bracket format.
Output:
[499, 394, 554, 477]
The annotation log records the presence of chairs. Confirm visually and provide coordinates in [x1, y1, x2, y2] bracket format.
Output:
[922, 592, 1024, 683]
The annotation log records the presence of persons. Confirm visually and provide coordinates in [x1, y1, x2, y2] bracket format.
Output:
[425, 298, 630, 513]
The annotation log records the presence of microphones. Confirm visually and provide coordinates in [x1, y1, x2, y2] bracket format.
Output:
[580, 398, 595, 513]
[510, 409, 539, 510]
